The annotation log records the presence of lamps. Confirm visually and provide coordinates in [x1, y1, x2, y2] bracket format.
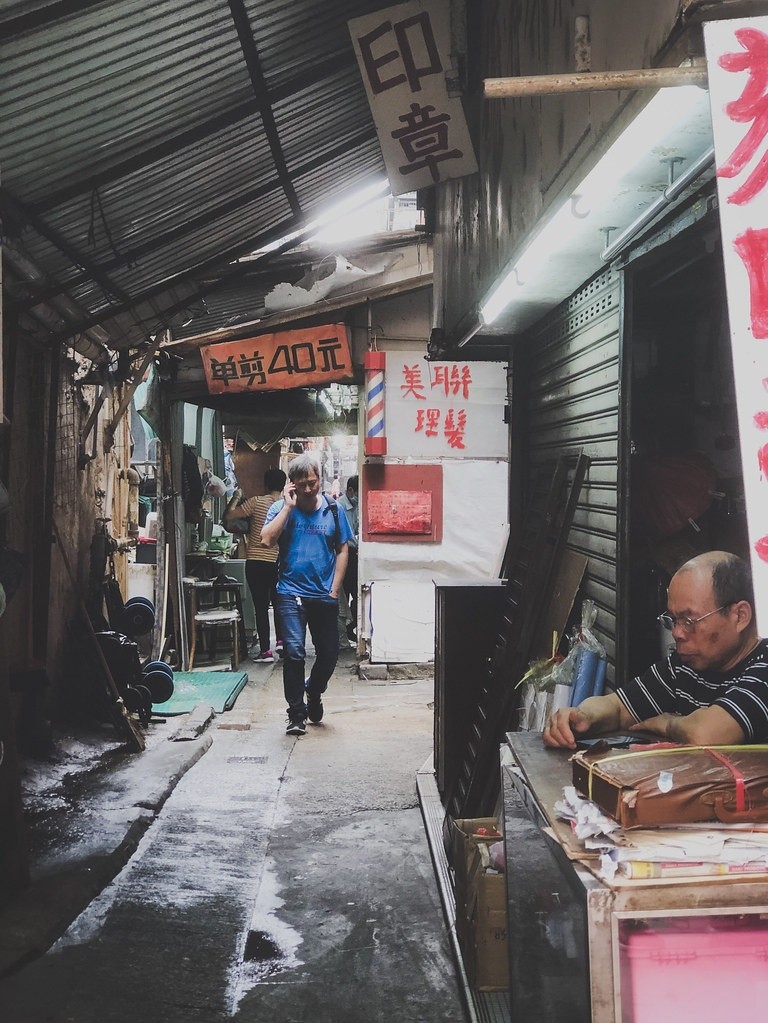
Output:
[363, 351, 387, 456]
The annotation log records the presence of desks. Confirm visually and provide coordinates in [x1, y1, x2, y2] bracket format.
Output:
[505, 728, 768, 1023]
[182, 582, 248, 666]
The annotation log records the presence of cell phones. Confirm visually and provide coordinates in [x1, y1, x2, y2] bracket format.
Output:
[290, 490, 294, 498]
[577, 730, 658, 750]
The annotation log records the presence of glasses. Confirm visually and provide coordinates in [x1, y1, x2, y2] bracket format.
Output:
[657, 601, 736, 634]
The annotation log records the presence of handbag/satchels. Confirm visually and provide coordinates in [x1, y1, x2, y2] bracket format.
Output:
[223, 494, 251, 534]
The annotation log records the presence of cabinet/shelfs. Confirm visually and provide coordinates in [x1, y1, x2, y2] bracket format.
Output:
[429, 577, 509, 805]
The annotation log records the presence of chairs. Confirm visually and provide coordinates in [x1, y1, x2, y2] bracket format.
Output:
[183, 578, 240, 672]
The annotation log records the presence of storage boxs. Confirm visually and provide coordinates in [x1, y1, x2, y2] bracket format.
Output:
[619, 920, 768, 1023]
[449, 816, 511, 994]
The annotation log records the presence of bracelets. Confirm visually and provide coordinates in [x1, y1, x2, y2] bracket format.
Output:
[231, 496, 240, 500]
[329, 590, 339, 596]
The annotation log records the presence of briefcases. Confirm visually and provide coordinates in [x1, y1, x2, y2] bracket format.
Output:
[571, 737, 768, 829]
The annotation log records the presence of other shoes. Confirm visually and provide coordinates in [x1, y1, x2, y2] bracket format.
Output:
[285, 718, 306, 734]
[253, 649, 275, 663]
[276, 640, 285, 658]
[306, 689, 323, 724]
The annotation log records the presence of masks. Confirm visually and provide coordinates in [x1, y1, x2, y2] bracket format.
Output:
[350, 488, 358, 505]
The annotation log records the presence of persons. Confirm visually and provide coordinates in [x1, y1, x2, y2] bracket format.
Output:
[223, 467, 286, 663]
[259, 457, 349, 736]
[330, 474, 360, 641]
[542, 552, 767, 749]
